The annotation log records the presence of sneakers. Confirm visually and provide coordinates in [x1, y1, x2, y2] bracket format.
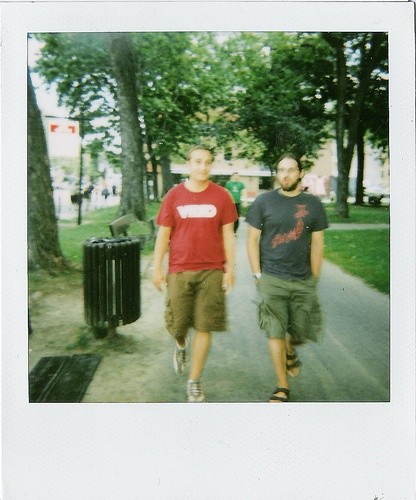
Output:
[174, 348, 187, 369]
[185, 379, 205, 402]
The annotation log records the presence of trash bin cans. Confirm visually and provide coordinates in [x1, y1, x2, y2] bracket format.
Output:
[80, 236, 141, 327]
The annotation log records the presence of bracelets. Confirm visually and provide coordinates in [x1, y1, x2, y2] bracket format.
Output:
[254, 273, 261, 280]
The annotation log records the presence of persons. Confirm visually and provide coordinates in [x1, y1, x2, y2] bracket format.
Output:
[224, 172, 245, 236]
[152, 145, 239, 403]
[245, 154, 328, 402]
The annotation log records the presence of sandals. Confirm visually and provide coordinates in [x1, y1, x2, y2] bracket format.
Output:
[285, 348, 302, 378]
[270, 387, 289, 403]
